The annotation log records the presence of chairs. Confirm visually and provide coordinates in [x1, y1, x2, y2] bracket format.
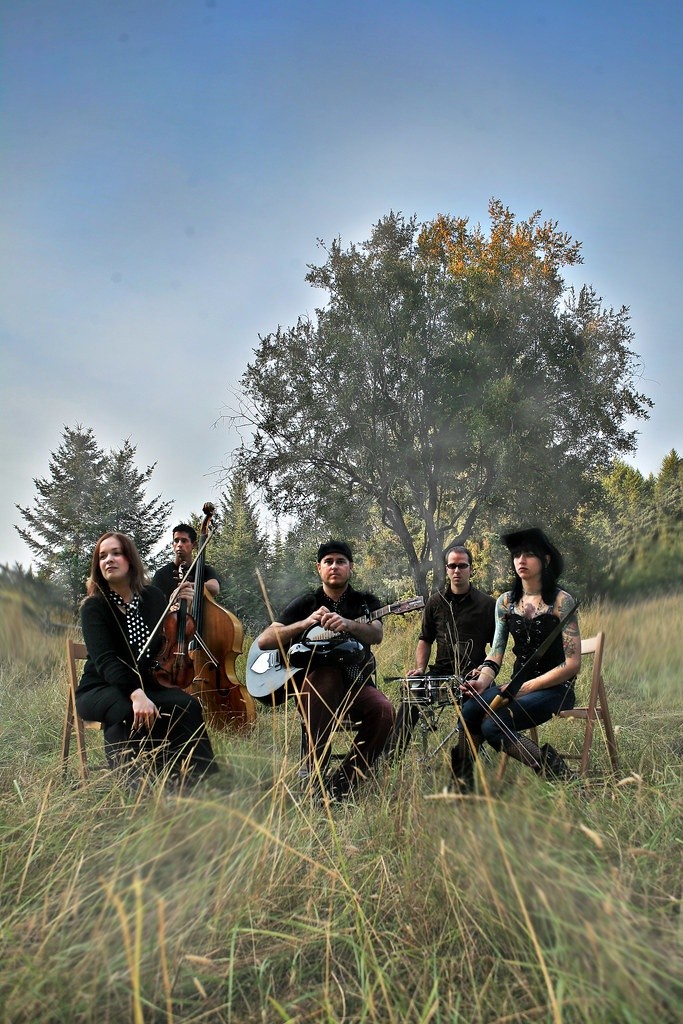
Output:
[61, 639, 110, 790]
[299, 716, 378, 788]
[495, 633, 619, 789]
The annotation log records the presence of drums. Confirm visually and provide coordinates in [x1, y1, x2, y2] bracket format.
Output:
[398, 677, 465, 703]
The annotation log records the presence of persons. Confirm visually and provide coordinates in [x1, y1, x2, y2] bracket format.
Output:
[380, 546, 497, 772]
[75, 532, 221, 788]
[257, 542, 396, 809]
[448, 528, 581, 795]
[151, 522, 224, 604]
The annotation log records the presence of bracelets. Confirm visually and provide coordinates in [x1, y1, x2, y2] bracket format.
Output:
[482, 660, 500, 680]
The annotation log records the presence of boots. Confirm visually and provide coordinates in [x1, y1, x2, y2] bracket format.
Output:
[533, 743, 596, 803]
[448, 748, 475, 795]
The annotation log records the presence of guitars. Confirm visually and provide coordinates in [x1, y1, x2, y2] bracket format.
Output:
[245, 596, 427, 706]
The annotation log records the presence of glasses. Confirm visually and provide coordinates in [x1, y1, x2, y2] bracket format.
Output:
[447, 563, 470, 569]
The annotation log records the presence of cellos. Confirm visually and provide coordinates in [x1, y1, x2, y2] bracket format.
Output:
[187, 501, 257, 738]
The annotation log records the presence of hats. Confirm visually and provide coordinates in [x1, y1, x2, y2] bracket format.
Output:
[503, 527, 564, 581]
[318, 541, 353, 563]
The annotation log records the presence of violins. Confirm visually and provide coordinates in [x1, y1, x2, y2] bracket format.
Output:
[151, 559, 196, 690]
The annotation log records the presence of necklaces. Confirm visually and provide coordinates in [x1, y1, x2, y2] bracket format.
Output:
[520, 589, 542, 645]
